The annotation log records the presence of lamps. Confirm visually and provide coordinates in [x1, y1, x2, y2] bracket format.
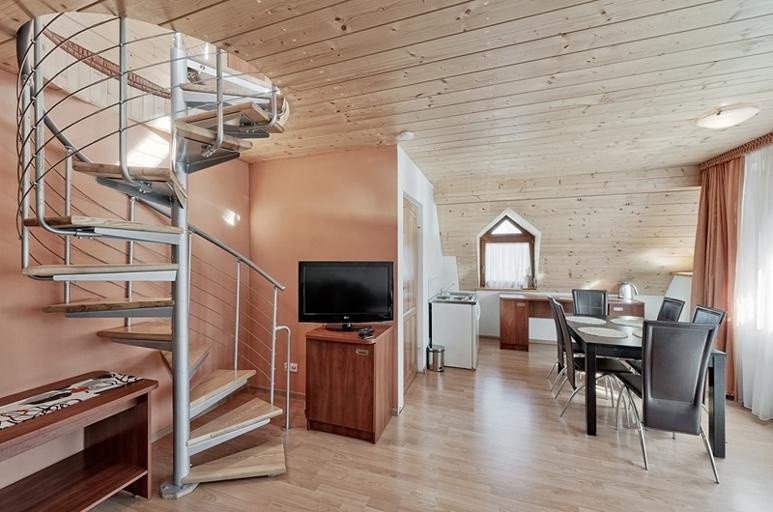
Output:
[697, 105, 761, 130]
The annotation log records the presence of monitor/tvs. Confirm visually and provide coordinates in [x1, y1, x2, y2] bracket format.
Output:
[297, 259, 395, 332]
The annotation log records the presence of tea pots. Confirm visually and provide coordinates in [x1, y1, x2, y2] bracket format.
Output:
[618, 282, 638, 299]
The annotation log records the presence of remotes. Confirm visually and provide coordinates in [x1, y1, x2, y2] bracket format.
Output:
[358, 326, 376, 335]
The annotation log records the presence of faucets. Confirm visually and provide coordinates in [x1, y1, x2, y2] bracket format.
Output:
[441, 283, 455, 294]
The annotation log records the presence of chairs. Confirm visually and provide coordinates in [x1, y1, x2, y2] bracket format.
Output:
[544, 289, 726, 484]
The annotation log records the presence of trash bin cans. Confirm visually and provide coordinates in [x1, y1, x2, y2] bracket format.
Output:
[427, 345, 444, 373]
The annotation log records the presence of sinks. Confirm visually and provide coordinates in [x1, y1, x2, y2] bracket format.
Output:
[448, 292, 474, 296]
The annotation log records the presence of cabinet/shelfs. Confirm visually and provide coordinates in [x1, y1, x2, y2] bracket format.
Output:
[0, 371, 159, 512]
[498, 292, 645, 352]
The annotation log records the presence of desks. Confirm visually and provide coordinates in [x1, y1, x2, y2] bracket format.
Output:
[304, 323, 394, 444]
